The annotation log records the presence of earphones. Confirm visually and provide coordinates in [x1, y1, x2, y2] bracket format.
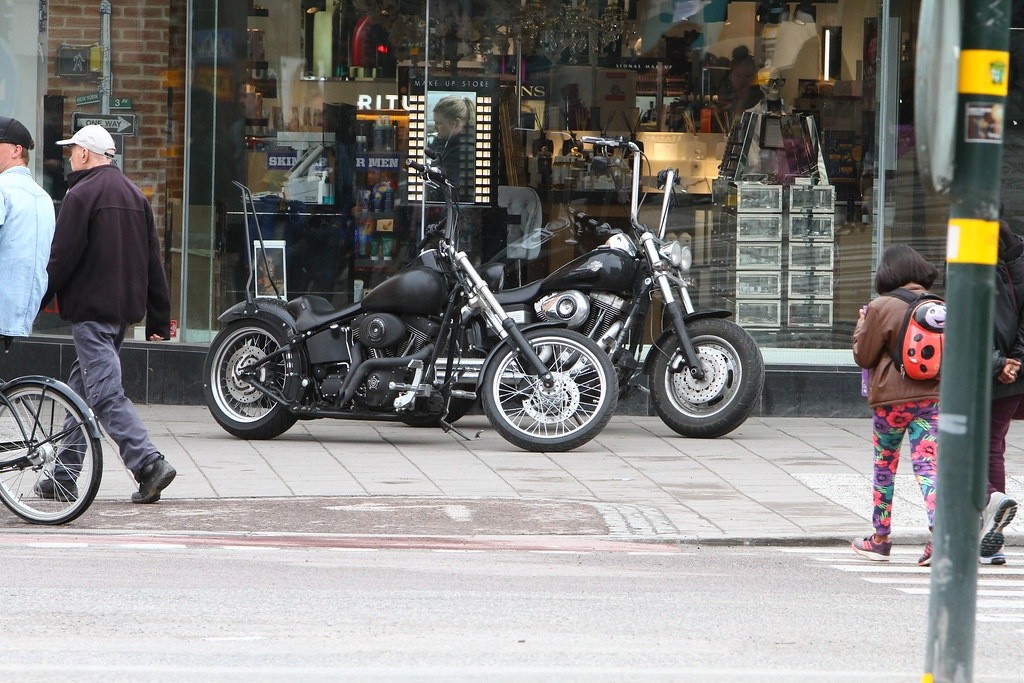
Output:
[453, 122, 456, 128]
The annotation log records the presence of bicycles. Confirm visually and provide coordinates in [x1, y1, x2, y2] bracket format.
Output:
[0, 335, 103, 526]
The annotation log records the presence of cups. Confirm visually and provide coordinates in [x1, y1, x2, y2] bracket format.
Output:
[356, 136, 367, 152]
[354, 279, 364, 302]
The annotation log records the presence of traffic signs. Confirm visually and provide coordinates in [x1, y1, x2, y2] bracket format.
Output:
[70, 112, 135, 135]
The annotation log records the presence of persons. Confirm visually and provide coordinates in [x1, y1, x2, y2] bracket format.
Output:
[567, 147, 583, 157]
[978, 199, 1024, 564]
[425, 96, 476, 202]
[0, 117, 57, 339]
[38, 123, 175, 504]
[537, 146, 551, 157]
[850, 243, 941, 566]
[718, 64, 830, 186]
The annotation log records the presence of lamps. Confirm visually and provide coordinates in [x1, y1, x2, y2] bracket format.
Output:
[755, 0, 790, 24]
[703, 0, 727, 22]
[791, 0, 812, 25]
[676, 0, 694, 21]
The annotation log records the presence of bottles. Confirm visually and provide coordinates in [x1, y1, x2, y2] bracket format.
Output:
[358, 114, 397, 261]
[271, 106, 322, 133]
[669, 94, 722, 132]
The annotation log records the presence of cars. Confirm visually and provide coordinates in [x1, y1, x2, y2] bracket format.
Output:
[245, 136, 271, 152]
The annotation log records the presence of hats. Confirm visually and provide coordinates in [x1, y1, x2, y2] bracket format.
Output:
[55, 125, 117, 159]
[0, 116, 34, 150]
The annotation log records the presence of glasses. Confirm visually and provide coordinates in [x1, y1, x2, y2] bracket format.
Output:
[760, 78, 785, 87]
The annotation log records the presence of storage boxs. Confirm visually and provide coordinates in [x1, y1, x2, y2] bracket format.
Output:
[714, 180, 834, 329]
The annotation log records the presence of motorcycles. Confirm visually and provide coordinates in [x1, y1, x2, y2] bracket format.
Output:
[201, 148, 619, 452]
[421, 136, 765, 439]
[476, 205, 622, 293]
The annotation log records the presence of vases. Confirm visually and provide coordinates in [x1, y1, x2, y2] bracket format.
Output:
[715, 133, 730, 173]
[685, 136, 706, 176]
[592, 133, 614, 159]
[532, 132, 554, 157]
[562, 134, 583, 156]
[622, 134, 644, 180]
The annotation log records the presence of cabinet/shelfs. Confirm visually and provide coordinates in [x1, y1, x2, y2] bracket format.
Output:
[246, 8, 269, 151]
[356, 109, 410, 296]
[796, 91, 868, 231]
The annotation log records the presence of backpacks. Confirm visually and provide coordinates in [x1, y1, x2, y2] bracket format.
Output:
[882, 288, 947, 384]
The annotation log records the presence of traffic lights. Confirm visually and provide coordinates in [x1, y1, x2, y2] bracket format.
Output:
[375, 43, 388, 66]
[55, 48, 90, 78]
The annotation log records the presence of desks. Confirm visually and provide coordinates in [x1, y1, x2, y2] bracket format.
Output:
[276, 132, 336, 177]
[527, 176, 713, 341]
[222, 199, 347, 329]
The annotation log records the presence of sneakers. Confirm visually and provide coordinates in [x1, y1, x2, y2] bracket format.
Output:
[852, 533, 892, 560]
[918, 541, 933, 566]
[131, 457, 176, 503]
[34, 478, 79, 501]
[978, 552, 1006, 565]
[979, 492, 1018, 558]
